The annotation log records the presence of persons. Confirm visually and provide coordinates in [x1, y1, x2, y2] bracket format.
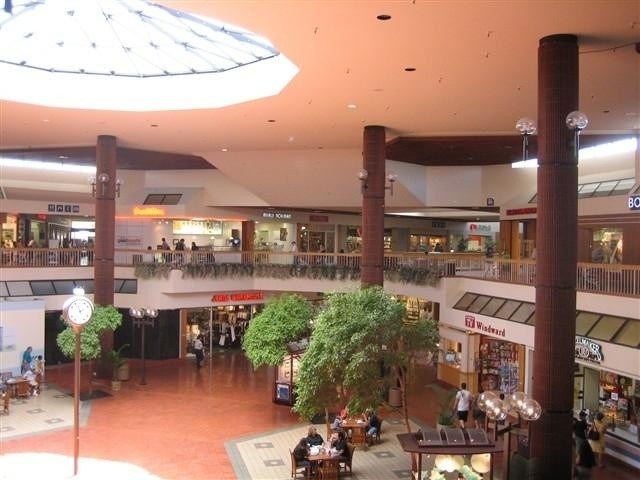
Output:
[35, 356, 48, 376]
[161, 238, 199, 264]
[572, 411, 591, 456]
[294, 407, 379, 477]
[344, 240, 354, 253]
[434, 242, 444, 252]
[470, 388, 487, 430]
[455, 239, 467, 252]
[574, 440, 598, 480]
[452, 383, 473, 429]
[1, 237, 94, 265]
[258, 238, 278, 250]
[23, 367, 39, 397]
[484, 241, 496, 277]
[142, 246, 158, 262]
[352, 242, 361, 266]
[289, 241, 301, 264]
[193, 335, 203, 369]
[496, 392, 507, 425]
[22, 347, 35, 374]
[315, 241, 348, 266]
[589, 412, 606, 468]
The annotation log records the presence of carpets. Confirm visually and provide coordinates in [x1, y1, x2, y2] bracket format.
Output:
[0, 383, 92, 442]
[223, 402, 436, 480]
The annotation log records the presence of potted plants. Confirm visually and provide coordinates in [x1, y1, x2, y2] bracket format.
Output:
[107, 343, 130, 390]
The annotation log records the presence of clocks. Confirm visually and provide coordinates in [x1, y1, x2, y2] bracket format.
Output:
[63, 296, 95, 326]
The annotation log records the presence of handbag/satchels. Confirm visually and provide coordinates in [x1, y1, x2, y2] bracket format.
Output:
[588, 422, 599, 440]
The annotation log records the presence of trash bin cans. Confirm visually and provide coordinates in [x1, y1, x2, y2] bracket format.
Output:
[447, 263, 455, 275]
[116, 362, 129, 380]
[133, 254, 143, 266]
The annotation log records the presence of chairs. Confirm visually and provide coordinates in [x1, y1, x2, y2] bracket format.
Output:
[287, 407, 384, 480]
[0, 371, 43, 413]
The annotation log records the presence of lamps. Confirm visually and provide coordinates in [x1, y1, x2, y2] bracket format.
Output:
[356, 168, 398, 197]
[515, 110, 589, 168]
[88, 171, 124, 197]
[476, 390, 542, 479]
